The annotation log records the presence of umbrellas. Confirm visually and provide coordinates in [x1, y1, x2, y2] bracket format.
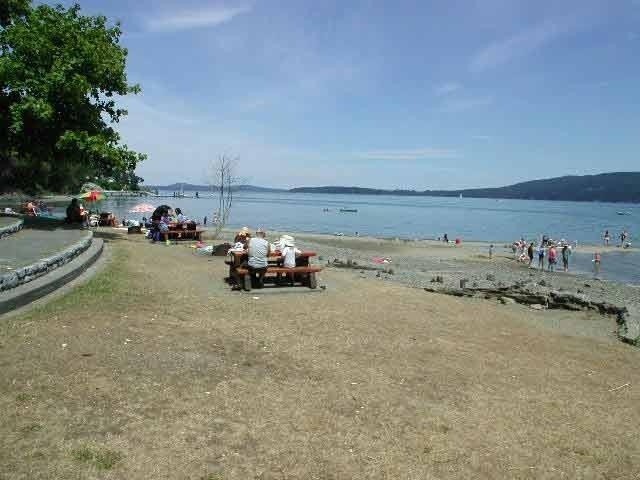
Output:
[77, 191, 111, 227]
[128, 203, 157, 228]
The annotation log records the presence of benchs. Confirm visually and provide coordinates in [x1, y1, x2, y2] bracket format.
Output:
[225, 260, 322, 290]
[148, 228, 205, 241]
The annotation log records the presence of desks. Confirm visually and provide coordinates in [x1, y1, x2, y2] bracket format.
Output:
[231, 249, 317, 283]
[149, 220, 200, 240]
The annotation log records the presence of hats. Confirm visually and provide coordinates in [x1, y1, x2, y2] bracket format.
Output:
[283, 235, 296, 247]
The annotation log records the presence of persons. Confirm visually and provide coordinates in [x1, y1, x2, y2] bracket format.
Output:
[489, 245, 494, 259]
[24, 199, 40, 217]
[233, 226, 301, 289]
[509, 229, 570, 272]
[592, 251, 601, 265]
[603, 229, 632, 249]
[67, 197, 117, 228]
[149, 204, 190, 246]
